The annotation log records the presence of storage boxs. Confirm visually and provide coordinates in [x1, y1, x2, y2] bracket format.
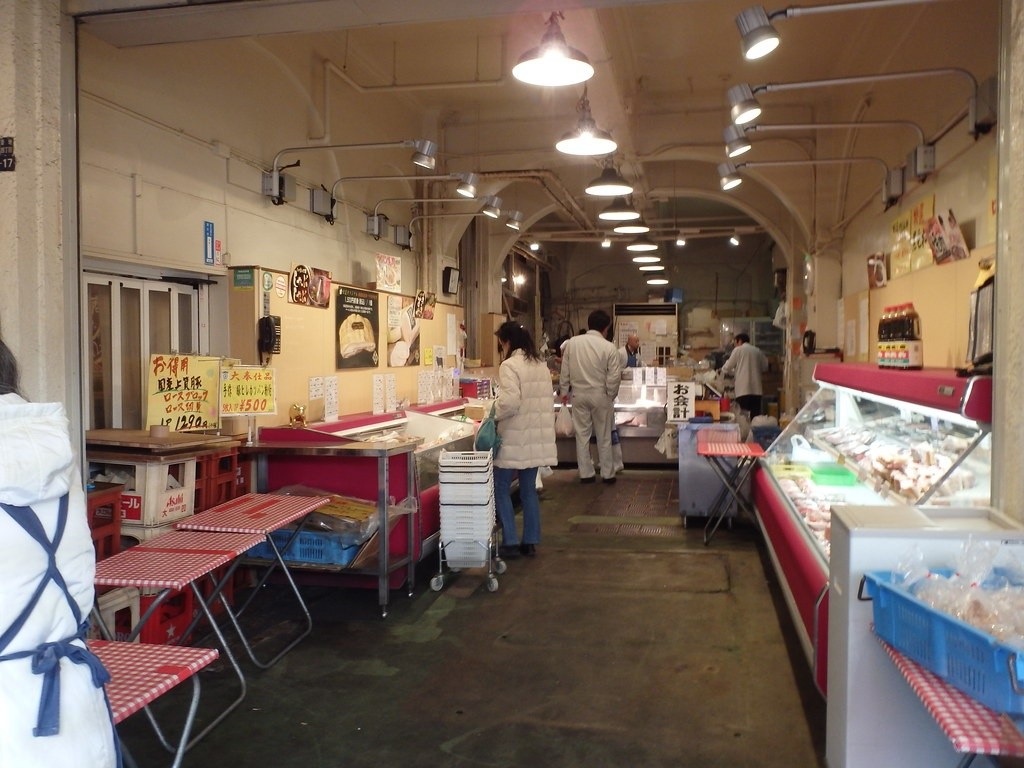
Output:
[88, 447, 252, 646]
[247, 528, 362, 565]
[857, 566, 1023, 717]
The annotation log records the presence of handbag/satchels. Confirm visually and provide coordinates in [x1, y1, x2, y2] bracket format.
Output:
[555, 403, 574, 438]
[475, 401, 502, 459]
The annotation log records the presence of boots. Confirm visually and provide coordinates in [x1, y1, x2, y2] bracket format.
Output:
[597, 443, 624, 471]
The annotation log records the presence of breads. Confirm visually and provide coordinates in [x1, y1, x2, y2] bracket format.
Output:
[387, 306, 420, 367]
[340, 313, 375, 357]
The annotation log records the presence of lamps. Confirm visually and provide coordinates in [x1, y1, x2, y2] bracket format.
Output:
[262, 0, 1000, 286]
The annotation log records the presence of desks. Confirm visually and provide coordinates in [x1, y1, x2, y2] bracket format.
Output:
[168, 493, 331, 670]
[869, 622, 1024, 768]
[83, 638, 219, 768]
[90, 530, 266, 755]
[696, 442, 766, 546]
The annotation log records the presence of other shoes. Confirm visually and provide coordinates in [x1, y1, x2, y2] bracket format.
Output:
[580, 477, 596, 482]
[491, 545, 521, 558]
[520, 543, 535, 556]
[603, 478, 616, 484]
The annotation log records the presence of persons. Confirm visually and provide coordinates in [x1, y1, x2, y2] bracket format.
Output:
[0, 342, 123, 768]
[721, 333, 769, 419]
[481, 322, 557, 558]
[560, 310, 621, 483]
[618, 334, 642, 368]
[595, 415, 624, 472]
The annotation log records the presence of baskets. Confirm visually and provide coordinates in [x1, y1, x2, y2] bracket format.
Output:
[438, 447, 496, 567]
[863, 566, 1024, 715]
[247, 530, 361, 565]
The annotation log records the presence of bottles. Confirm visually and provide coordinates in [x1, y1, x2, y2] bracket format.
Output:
[877, 302, 924, 370]
